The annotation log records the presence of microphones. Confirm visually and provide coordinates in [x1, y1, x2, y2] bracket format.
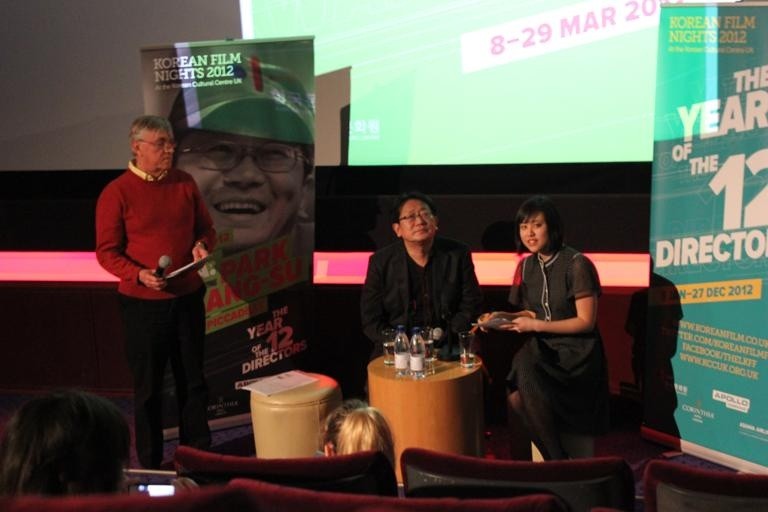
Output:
[432, 327, 443, 349]
[154, 255, 172, 277]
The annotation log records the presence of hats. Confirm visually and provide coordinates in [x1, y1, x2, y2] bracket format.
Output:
[166, 59, 316, 149]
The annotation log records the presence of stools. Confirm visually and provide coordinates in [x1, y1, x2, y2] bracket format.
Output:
[249, 372, 346, 461]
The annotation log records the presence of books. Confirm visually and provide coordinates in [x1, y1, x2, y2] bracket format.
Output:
[240, 369, 320, 396]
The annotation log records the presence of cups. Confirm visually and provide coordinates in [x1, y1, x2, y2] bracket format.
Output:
[381, 328, 399, 366]
[420, 325, 436, 363]
[459, 330, 477, 369]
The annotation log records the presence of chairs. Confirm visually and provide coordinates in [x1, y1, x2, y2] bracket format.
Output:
[640, 456, 766, 510]
[226, 476, 573, 511]
[172, 442, 399, 497]
[1, 484, 248, 511]
[400, 445, 637, 511]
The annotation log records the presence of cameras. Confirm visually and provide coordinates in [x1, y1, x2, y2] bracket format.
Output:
[129, 483, 175, 497]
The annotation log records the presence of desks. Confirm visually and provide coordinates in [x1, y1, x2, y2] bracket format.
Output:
[366, 350, 486, 487]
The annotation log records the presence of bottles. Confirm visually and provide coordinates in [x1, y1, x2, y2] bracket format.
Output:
[408, 328, 427, 382]
[393, 325, 411, 376]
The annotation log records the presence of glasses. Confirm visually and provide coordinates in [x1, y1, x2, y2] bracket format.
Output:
[178, 140, 307, 173]
[141, 138, 177, 149]
[397, 210, 433, 221]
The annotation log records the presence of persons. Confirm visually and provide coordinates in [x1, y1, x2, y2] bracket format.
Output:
[0, 389, 200, 498]
[167, 57, 314, 253]
[320, 397, 397, 484]
[359, 191, 482, 357]
[475, 193, 611, 461]
[93, 113, 217, 469]
